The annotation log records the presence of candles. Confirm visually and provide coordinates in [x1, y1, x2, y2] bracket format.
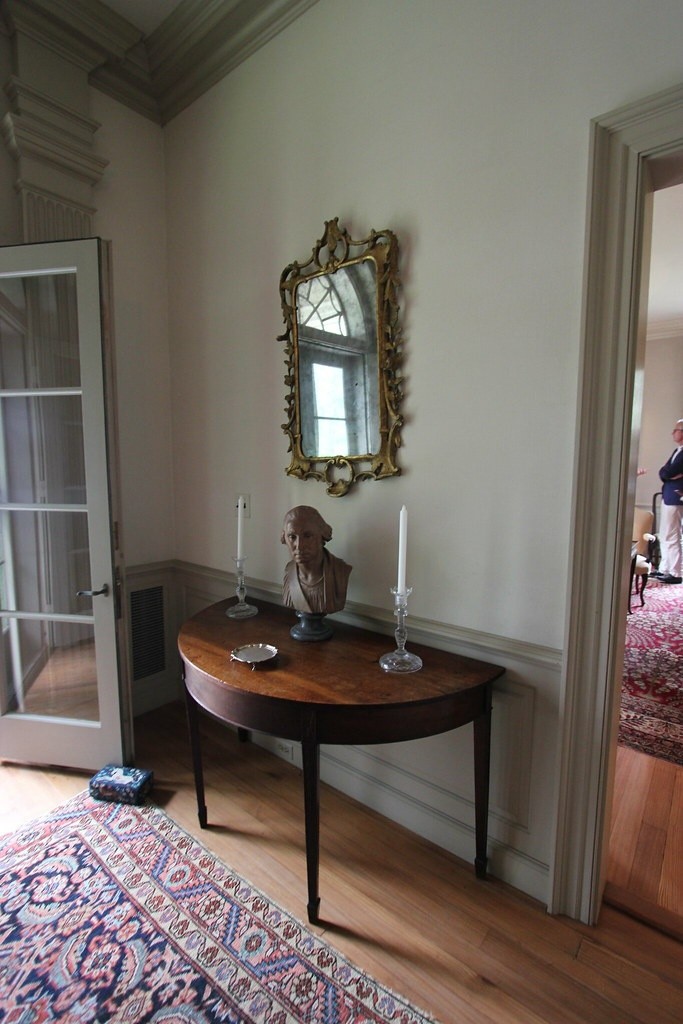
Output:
[237, 495, 245, 558]
[397, 504, 408, 595]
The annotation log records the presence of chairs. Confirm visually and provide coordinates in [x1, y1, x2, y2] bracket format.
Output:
[631, 506, 654, 607]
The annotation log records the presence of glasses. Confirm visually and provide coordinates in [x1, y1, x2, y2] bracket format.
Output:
[672, 429, 682, 434]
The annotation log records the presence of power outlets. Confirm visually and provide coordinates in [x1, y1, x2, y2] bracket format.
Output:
[232, 493, 251, 518]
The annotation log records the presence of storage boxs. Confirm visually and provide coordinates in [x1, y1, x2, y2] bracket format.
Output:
[89, 764, 154, 805]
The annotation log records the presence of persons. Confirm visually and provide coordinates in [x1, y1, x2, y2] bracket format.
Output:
[280, 504, 354, 614]
[650, 418, 683, 583]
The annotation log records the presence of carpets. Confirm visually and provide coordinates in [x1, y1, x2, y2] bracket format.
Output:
[0, 788, 443, 1024]
[616, 564, 683, 766]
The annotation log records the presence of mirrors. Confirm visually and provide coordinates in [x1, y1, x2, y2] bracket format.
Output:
[275, 216, 406, 501]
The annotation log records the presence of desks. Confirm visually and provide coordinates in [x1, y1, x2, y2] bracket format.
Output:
[178, 594, 509, 925]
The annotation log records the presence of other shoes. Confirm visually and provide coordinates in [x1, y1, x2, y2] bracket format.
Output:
[650, 571, 663, 576]
[655, 574, 682, 584]
